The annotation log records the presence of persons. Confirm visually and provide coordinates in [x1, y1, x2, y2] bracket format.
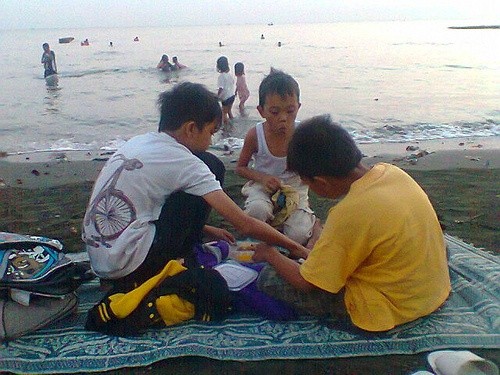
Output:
[82, 39, 89, 46]
[110, 42, 113, 47]
[157, 55, 172, 72]
[173, 57, 186, 68]
[41, 43, 57, 78]
[278, 41, 281, 47]
[216, 57, 236, 125]
[81, 83, 311, 292]
[250, 113, 452, 335]
[261, 34, 265, 39]
[234, 62, 250, 109]
[134, 37, 139, 41]
[235, 67, 317, 247]
[219, 42, 222, 46]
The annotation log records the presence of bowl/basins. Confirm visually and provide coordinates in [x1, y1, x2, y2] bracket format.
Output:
[231, 240, 259, 262]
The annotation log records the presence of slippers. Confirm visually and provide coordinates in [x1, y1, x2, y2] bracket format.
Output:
[428, 350, 499, 375]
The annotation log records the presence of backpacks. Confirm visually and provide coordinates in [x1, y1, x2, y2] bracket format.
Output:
[1, 232, 78, 297]
